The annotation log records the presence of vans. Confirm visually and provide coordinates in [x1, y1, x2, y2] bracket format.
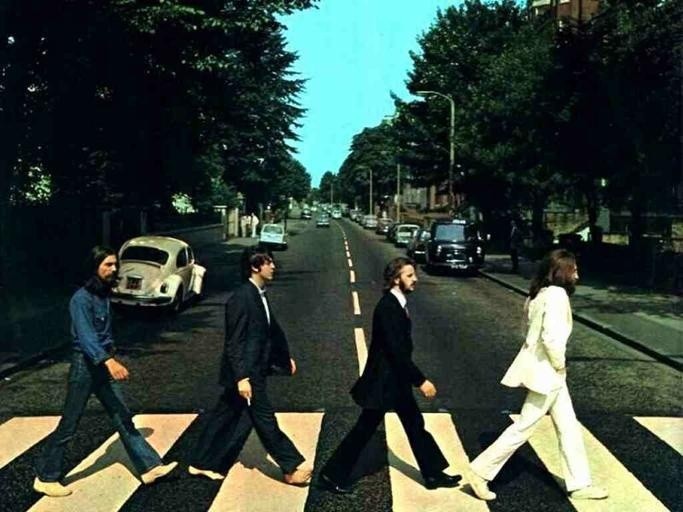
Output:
[258, 223, 289, 251]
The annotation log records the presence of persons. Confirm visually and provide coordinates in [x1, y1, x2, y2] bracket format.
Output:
[321, 257, 462, 494]
[465, 250, 609, 501]
[33, 245, 178, 496]
[188, 246, 313, 484]
[507, 220, 525, 270]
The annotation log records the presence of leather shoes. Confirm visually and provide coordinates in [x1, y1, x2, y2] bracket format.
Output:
[316, 470, 350, 497]
[424, 472, 462, 490]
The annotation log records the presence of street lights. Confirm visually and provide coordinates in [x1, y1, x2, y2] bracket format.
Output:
[416, 90, 456, 203]
[379, 151, 400, 223]
[357, 165, 372, 214]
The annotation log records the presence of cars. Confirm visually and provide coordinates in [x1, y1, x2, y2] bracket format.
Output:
[300, 199, 487, 278]
[105, 235, 208, 316]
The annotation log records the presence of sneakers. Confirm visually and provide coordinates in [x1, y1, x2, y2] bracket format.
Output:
[571, 486, 609, 500]
[465, 469, 497, 500]
[140, 461, 179, 485]
[31, 477, 73, 498]
